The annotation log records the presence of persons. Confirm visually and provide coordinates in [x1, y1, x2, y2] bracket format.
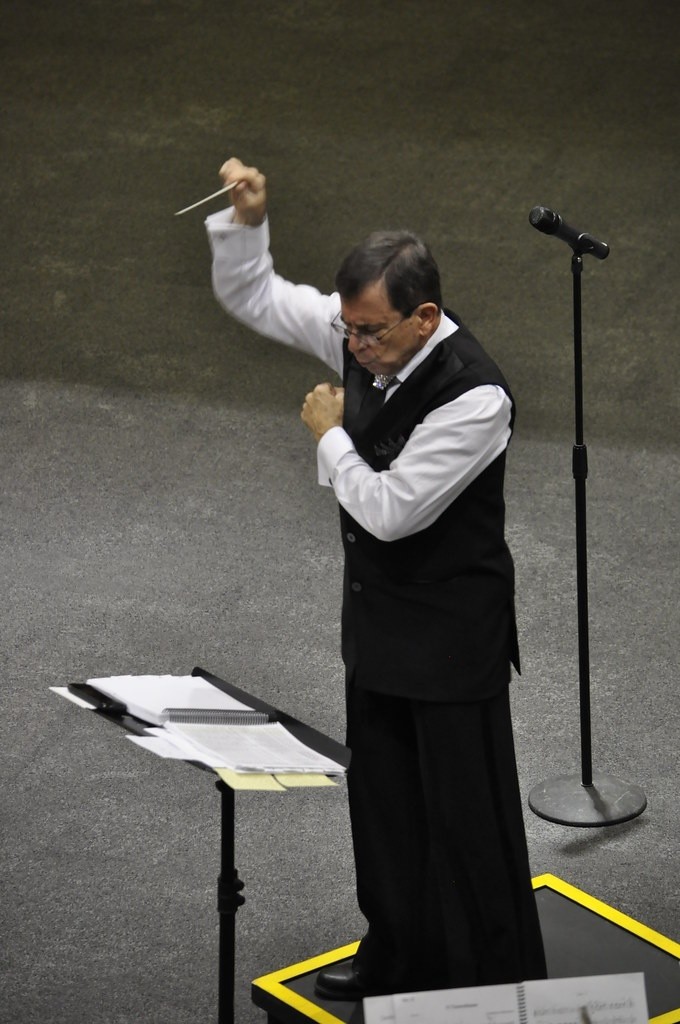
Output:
[200, 156, 547, 1023]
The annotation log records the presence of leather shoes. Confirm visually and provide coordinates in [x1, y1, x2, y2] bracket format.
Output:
[315, 958, 374, 1000]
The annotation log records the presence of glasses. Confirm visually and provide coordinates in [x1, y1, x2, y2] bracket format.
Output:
[330, 311, 407, 348]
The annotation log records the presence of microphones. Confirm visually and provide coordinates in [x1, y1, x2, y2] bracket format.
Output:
[528, 205, 610, 259]
